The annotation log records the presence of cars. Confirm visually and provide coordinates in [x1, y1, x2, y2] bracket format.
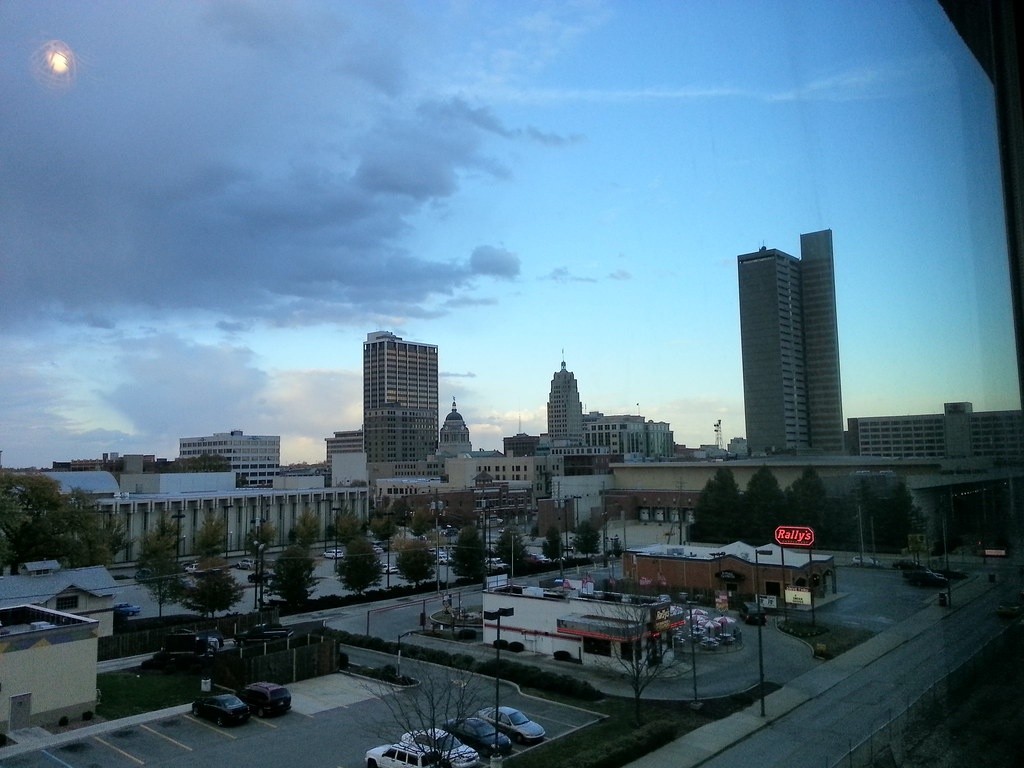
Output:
[236, 558, 256, 571]
[485, 557, 509, 571]
[372, 544, 384, 555]
[382, 563, 398, 575]
[113, 602, 141, 616]
[478, 706, 546, 744]
[418, 527, 459, 541]
[892, 558, 948, 587]
[401, 728, 480, 768]
[442, 717, 512, 759]
[531, 553, 567, 564]
[192, 693, 250, 727]
[428, 547, 453, 565]
[853, 555, 882, 568]
[185, 563, 200, 574]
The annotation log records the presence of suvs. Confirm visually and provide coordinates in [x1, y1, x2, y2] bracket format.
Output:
[323, 550, 343, 560]
[739, 601, 766, 626]
[237, 681, 292, 719]
[364, 740, 442, 768]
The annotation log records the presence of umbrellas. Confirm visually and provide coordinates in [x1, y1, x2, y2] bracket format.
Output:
[686, 609, 736, 642]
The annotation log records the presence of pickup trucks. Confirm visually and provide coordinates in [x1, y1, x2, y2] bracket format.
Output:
[233, 623, 295, 649]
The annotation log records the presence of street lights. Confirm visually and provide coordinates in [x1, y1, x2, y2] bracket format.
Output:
[686, 600, 703, 710]
[250, 518, 266, 613]
[755, 550, 773, 718]
[383, 510, 396, 589]
[573, 496, 582, 528]
[170, 509, 187, 562]
[484, 504, 494, 576]
[483, 606, 515, 768]
[221, 505, 233, 559]
[430, 499, 443, 597]
[331, 507, 342, 573]
